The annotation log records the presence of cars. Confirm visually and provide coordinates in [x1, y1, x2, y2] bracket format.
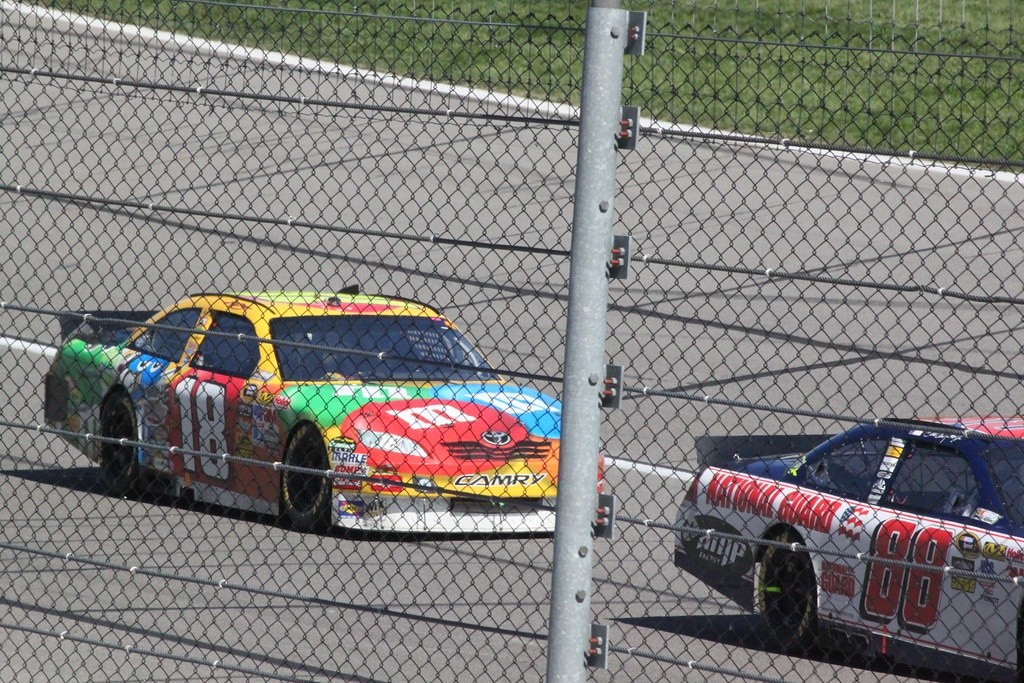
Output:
[674, 418, 1024, 683]
[43, 284, 602, 539]
